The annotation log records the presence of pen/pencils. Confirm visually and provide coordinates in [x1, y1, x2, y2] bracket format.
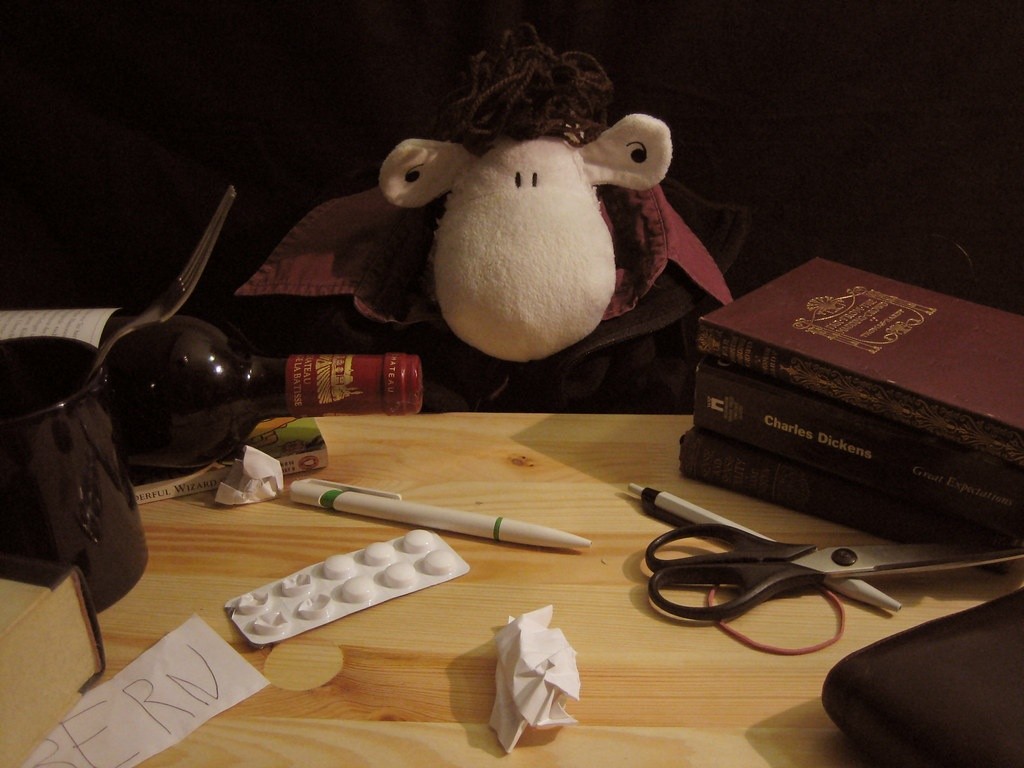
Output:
[629, 482, 903, 613]
[291, 477, 593, 548]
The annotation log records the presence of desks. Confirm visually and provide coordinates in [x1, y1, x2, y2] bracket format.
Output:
[0, 411, 1024, 768]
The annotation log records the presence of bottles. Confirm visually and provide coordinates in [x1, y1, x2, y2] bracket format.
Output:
[1, 310, 424, 484]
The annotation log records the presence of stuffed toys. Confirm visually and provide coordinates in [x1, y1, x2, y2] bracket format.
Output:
[237, 18, 736, 413]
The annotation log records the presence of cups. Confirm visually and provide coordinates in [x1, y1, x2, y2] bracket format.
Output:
[1, 337, 148, 613]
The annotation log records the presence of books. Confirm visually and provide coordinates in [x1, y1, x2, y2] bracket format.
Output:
[675, 256, 1024, 577]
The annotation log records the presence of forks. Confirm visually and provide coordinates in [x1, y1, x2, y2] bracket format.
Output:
[86, 184, 236, 380]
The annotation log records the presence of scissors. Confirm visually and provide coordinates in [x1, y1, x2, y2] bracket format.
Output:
[646, 522, 1024, 620]
